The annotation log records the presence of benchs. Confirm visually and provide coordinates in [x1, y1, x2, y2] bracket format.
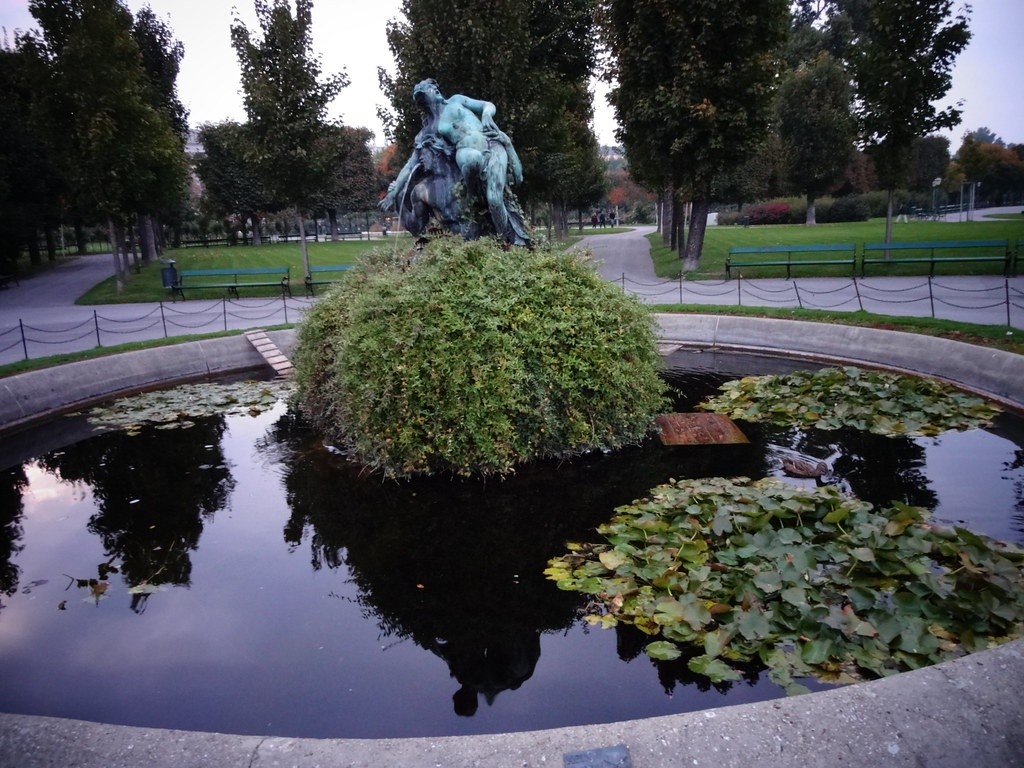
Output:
[860, 239, 1012, 279]
[724, 243, 856, 281]
[304, 264, 355, 299]
[170, 267, 292, 303]
[183, 232, 362, 248]
[0, 263, 20, 290]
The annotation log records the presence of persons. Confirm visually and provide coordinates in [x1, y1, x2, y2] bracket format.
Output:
[894, 202, 909, 225]
[376, 78, 532, 250]
[591, 210, 615, 228]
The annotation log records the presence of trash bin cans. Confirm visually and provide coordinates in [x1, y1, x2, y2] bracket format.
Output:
[160, 258, 179, 288]
[744, 216, 749, 226]
